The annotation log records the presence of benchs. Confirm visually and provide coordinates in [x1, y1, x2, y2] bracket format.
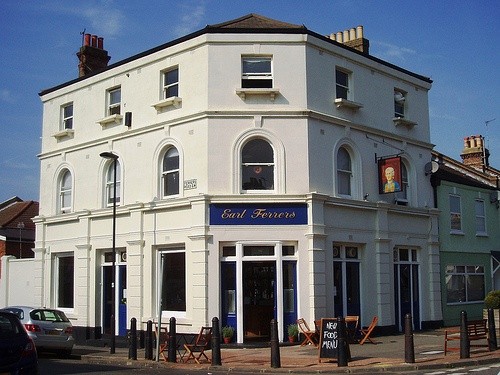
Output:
[441, 319, 489, 357]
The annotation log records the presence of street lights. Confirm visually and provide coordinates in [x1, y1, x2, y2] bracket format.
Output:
[99, 152, 121, 353]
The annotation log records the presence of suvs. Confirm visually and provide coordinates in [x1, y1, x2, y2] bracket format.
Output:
[0, 313, 40, 374]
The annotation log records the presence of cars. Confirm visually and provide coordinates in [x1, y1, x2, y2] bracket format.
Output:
[7, 306, 76, 356]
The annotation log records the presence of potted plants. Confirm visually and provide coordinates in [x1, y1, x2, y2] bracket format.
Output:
[221, 325, 235, 344]
[287, 322, 300, 345]
[482, 288, 500, 338]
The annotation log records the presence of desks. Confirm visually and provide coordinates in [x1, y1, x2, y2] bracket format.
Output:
[314, 319, 356, 345]
[141, 320, 193, 363]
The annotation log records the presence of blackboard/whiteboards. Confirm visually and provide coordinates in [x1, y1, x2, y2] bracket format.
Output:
[318, 317, 352, 361]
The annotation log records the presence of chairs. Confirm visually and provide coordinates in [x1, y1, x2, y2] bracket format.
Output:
[31, 315, 39, 320]
[344, 316, 359, 344]
[179, 326, 213, 365]
[298, 317, 319, 349]
[154, 323, 185, 362]
[357, 316, 378, 346]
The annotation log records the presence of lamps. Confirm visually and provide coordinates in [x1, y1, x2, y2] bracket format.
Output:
[125, 111, 132, 127]
